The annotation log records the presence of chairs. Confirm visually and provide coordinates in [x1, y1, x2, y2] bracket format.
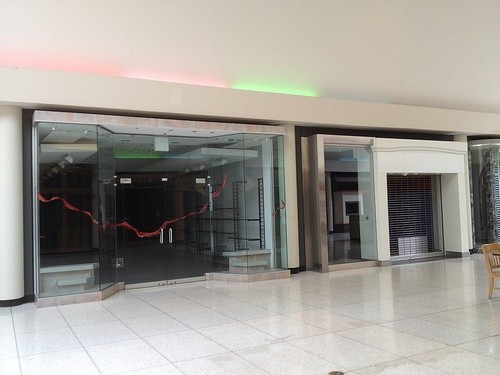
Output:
[481, 241, 500, 298]
